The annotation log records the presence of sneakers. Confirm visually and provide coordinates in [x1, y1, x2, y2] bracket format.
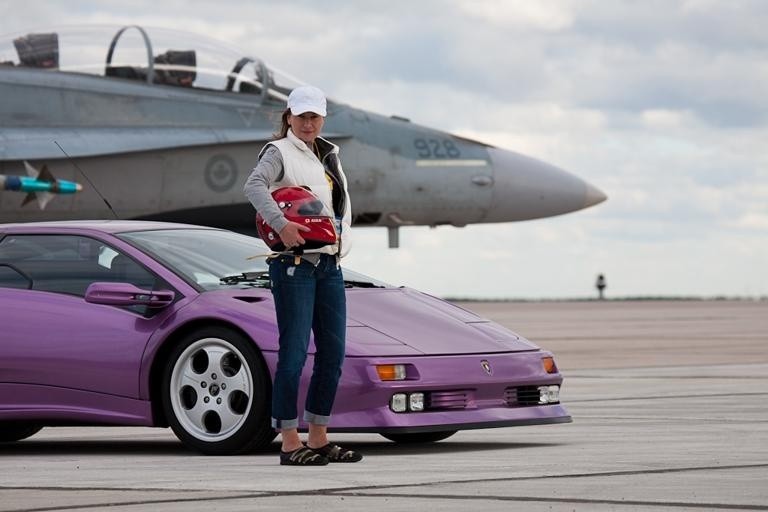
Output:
[280, 443, 362, 465]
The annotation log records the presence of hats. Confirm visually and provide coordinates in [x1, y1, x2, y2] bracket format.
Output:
[287, 86, 327, 117]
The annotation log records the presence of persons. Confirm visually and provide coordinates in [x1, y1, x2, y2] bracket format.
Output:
[243, 86, 365, 466]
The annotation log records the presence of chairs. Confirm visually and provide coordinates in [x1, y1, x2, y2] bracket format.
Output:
[111, 251, 197, 287]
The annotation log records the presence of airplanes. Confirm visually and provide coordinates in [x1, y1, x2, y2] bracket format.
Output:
[0, 21, 607, 249]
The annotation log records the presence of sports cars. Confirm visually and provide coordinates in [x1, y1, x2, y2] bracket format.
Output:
[1, 218, 574, 454]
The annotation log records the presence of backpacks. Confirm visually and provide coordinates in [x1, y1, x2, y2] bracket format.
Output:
[256, 186, 336, 255]
